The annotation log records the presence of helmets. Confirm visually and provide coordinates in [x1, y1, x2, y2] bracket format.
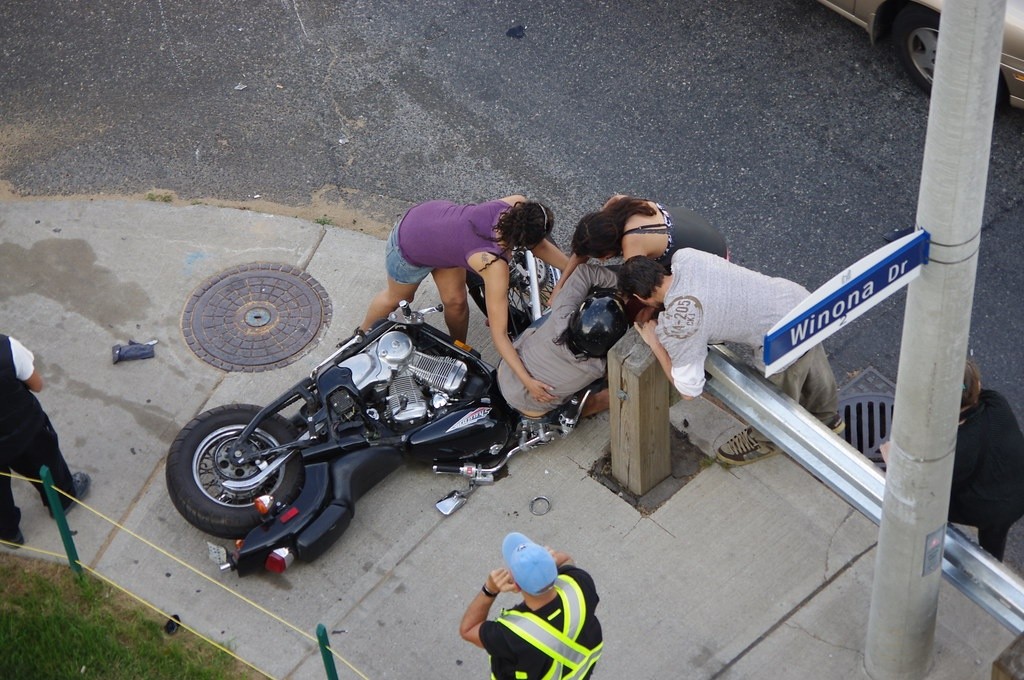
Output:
[570, 290, 631, 357]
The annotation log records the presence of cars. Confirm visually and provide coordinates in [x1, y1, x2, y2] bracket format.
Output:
[818, 0, 1024, 111]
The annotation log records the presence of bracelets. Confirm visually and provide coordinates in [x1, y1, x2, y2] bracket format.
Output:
[482, 585, 499, 598]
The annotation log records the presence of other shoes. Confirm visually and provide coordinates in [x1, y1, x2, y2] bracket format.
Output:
[4, 529, 24, 549]
[50, 471, 91, 518]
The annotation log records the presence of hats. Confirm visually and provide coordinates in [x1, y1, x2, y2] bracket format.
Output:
[501, 532, 559, 595]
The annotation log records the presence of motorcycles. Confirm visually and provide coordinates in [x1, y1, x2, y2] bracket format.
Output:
[165, 232, 604, 576]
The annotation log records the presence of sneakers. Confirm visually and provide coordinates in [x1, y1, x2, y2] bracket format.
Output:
[718, 428, 784, 464]
[828, 413, 845, 435]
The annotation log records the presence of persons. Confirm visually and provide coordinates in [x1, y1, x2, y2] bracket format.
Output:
[459, 530, 604, 680]
[548, 195, 729, 323]
[360, 194, 569, 404]
[0, 332, 90, 549]
[617, 248, 845, 466]
[947, 362, 1024, 563]
[497, 264, 629, 431]
